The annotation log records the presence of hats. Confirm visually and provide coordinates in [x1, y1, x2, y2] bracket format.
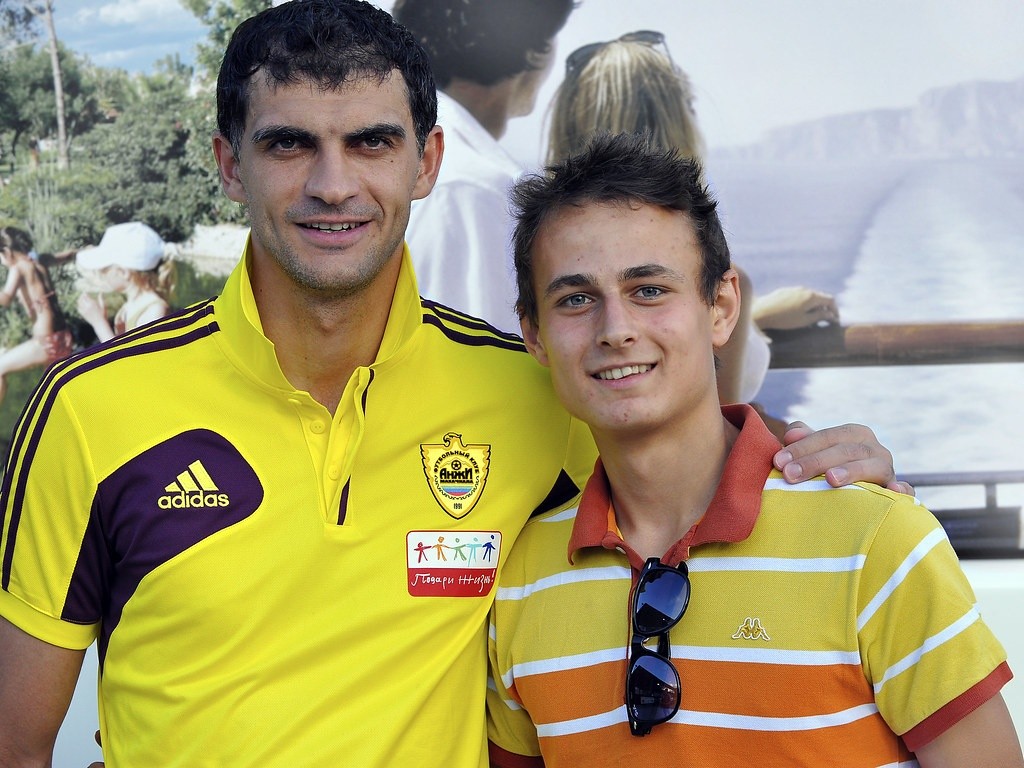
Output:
[75, 221, 163, 270]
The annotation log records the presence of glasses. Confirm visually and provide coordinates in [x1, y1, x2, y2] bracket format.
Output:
[565, 28, 680, 95]
[623, 554, 691, 738]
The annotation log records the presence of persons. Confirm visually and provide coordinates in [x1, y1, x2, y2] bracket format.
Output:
[0, 0, 917, 768]
[390, 1, 840, 405]
[1, 221, 179, 399]
[91, 127, 1023, 767]
